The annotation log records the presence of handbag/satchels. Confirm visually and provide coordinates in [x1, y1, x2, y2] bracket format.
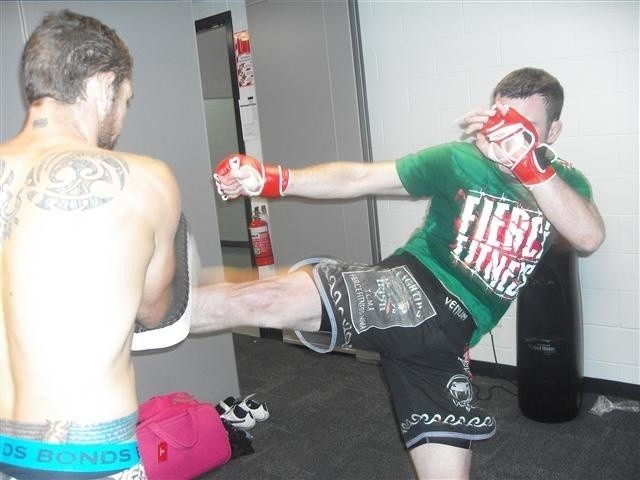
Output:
[134, 389, 233, 480]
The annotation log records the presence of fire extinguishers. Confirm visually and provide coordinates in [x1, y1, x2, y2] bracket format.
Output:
[248, 205, 274, 266]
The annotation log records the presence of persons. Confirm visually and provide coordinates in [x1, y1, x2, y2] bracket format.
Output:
[186, 67, 606, 480]
[1, 8, 184, 480]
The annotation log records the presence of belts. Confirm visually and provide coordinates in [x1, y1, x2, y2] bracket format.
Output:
[0, 408, 140, 444]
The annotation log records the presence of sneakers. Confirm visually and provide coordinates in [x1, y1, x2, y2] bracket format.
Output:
[215, 396, 257, 433]
[233, 391, 272, 424]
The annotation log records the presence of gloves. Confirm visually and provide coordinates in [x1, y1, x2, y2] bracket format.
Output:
[476, 104, 560, 194]
[212, 151, 292, 203]
